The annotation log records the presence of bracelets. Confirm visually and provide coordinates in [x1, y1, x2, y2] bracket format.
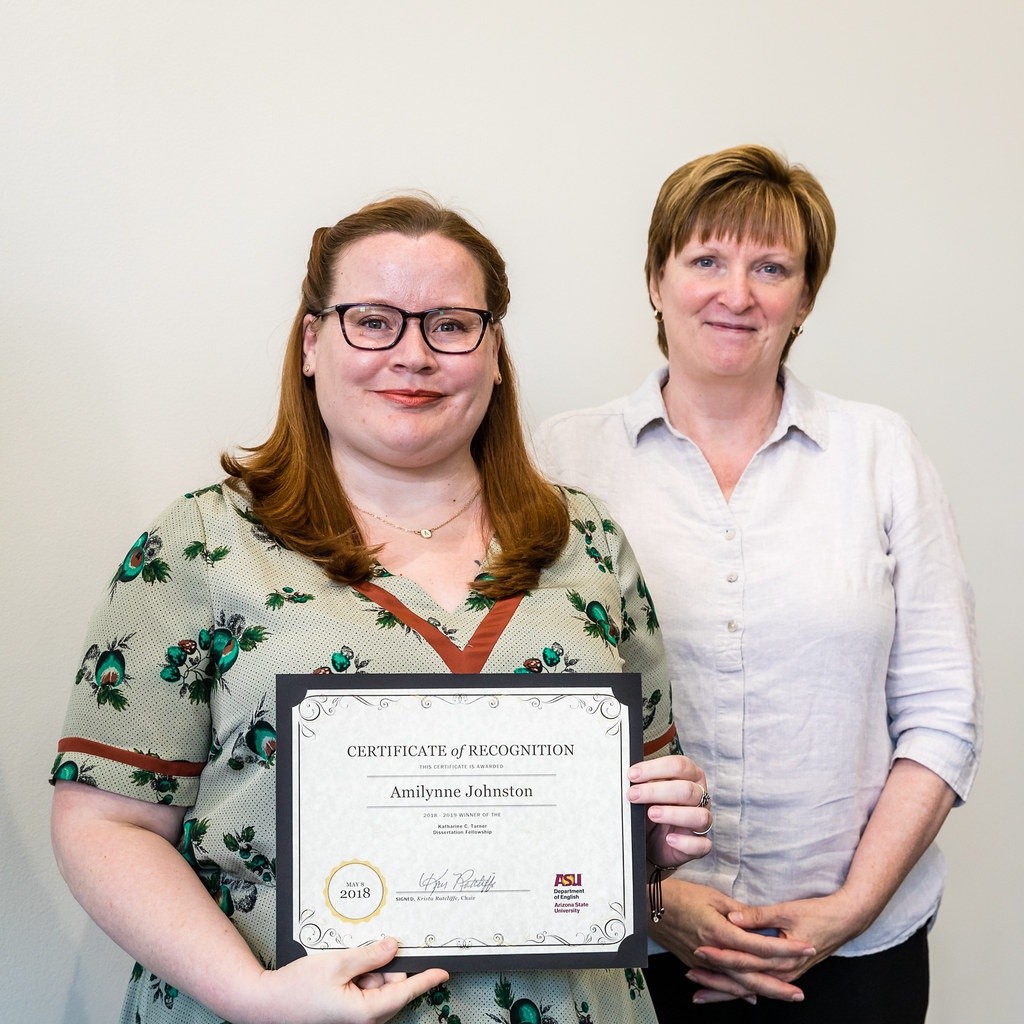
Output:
[646, 823, 679, 924]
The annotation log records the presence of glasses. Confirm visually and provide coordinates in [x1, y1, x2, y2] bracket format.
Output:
[317, 303, 493, 355]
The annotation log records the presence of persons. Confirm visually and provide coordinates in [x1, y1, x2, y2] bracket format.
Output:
[529, 144, 979, 1024]
[48, 192, 715, 1024]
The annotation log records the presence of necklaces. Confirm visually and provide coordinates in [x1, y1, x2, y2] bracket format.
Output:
[343, 488, 483, 540]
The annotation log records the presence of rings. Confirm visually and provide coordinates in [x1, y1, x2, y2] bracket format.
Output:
[697, 786, 709, 808]
[692, 821, 714, 835]
